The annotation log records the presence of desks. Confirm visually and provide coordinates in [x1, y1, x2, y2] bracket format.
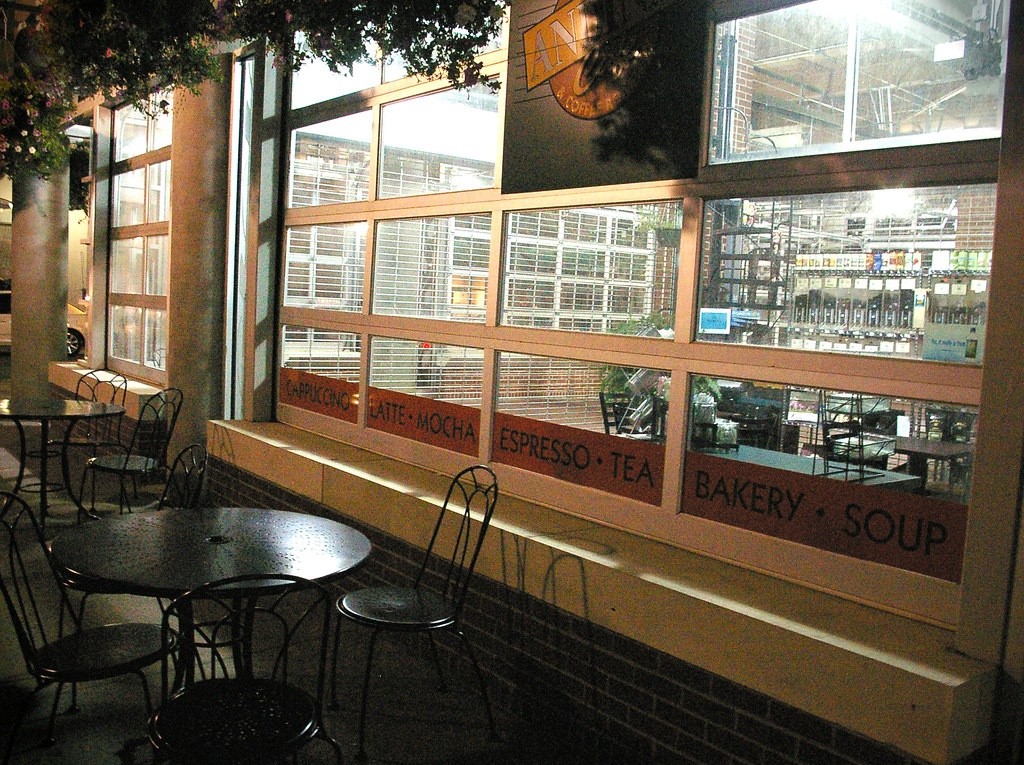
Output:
[857, 435, 975, 491]
[0, 399, 126, 524]
[44, 508, 371, 765]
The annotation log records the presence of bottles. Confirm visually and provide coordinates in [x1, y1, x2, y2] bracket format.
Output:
[794, 297, 986, 329]
[964, 327, 978, 363]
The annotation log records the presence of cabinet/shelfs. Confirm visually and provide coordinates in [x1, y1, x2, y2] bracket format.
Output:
[652, 394, 739, 451]
[810, 387, 906, 486]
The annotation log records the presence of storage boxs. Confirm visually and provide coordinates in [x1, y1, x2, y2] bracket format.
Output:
[826, 433, 896, 459]
[827, 394, 892, 414]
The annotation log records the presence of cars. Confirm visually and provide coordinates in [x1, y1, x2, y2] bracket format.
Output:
[0, 276, 90, 360]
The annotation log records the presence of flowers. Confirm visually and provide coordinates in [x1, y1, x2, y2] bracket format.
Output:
[0, 0, 503, 172]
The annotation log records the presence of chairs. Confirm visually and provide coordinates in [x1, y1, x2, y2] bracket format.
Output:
[76, 386, 184, 524]
[143, 572, 343, 765]
[47, 369, 127, 508]
[598, 391, 641, 435]
[55, 444, 207, 637]
[329, 464, 501, 762]
[0, 491, 177, 765]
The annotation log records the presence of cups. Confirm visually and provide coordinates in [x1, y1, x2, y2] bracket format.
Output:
[628, 395, 662, 432]
[628, 367, 660, 396]
[637, 326, 662, 338]
